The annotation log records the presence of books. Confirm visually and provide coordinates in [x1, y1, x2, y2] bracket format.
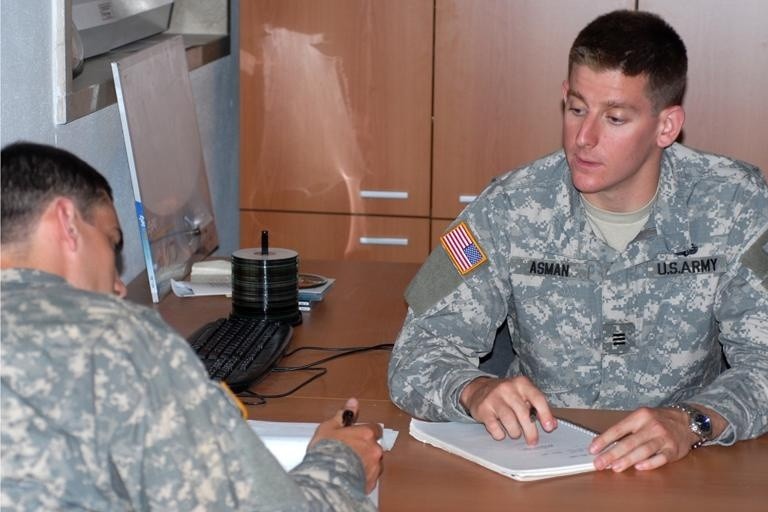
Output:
[249, 411, 382, 512]
[403, 409, 647, 481]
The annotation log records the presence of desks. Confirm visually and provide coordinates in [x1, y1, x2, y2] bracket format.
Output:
[128, 256, 768, 512]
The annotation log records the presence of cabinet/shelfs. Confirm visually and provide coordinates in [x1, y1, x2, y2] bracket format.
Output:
[237, 0, 768, 264]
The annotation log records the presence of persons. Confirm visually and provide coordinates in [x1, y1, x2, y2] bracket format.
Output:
[377, 7, 768, 484]
[1, 136, 385, 511]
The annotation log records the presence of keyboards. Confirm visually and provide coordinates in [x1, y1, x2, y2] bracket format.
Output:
[180, 315, 294, 394]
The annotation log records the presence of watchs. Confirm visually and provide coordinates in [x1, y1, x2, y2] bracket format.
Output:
[655, 398, 714, 455]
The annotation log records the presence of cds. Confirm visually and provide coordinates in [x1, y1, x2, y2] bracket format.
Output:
[230, 246, 301, 327]
[298, 270, 330, 288]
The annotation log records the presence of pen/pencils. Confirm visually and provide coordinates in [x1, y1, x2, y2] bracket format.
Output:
[342, 409, 354, 427]
